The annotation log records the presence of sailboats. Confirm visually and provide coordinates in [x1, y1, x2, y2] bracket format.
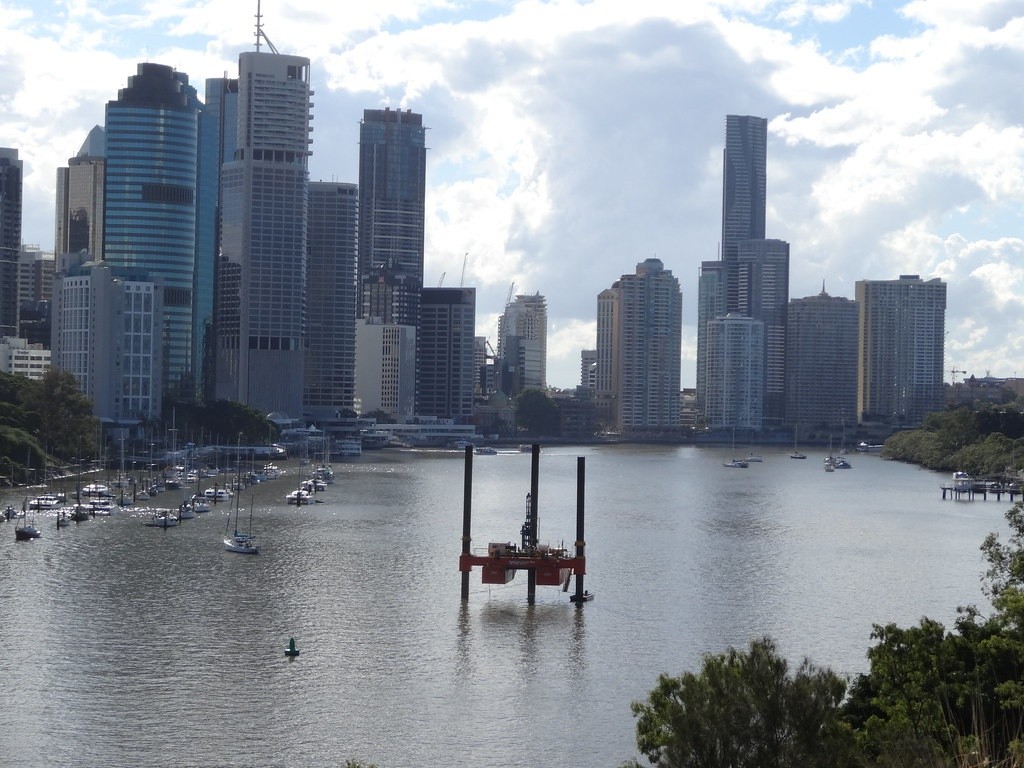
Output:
[723, 427, 764, 468]
[222, 429, 258, 556]
[789, 424, 807, 459]
[0, 405, 334, 540]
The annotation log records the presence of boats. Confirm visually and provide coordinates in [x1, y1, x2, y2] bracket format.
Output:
[824, 432, 851, 472]
[339, 434, 362, 456]
[857, 441, 869, 453]
[473, 446, 498, 456]
[519, 444, 542, 453]
[952, 471, 974, 491]
